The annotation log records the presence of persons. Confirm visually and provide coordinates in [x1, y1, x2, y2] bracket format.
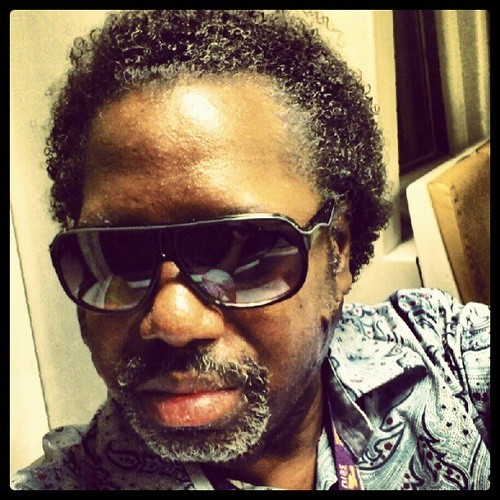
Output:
[9, 9, 495, 493]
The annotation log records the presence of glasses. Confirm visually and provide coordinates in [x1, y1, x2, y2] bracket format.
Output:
[49, 197, 336, 315]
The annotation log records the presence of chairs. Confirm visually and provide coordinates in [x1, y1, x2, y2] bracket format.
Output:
[403, 135, 491, 312]
